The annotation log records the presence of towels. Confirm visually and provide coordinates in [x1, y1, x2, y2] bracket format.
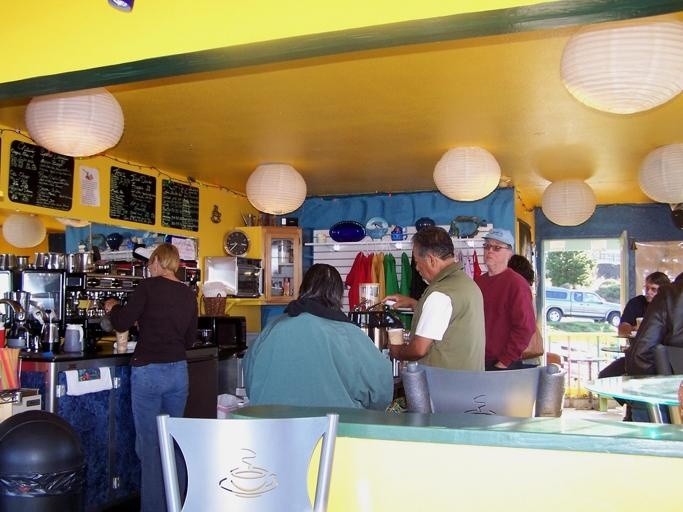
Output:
[63, 367, 114, 396]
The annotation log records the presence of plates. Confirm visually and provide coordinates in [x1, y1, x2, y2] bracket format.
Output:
[366, 217, 388, 238]
[329, 221, 366, 242]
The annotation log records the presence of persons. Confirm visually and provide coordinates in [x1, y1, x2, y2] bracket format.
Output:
[475, 228, 536, 371]
[104, 244, 198, 512]
[507, 254, 545, 369]
[598, 271, 671, 421]
[386, 225, 487, 372]
[240, 262, 394, 411]
[628, 272, 683, 376]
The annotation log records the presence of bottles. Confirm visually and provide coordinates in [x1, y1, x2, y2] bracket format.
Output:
[283, 278, 290, 297]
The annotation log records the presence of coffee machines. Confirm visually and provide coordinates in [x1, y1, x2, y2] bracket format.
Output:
[64, 324, 84, 352]
[348, 305, 402, 377]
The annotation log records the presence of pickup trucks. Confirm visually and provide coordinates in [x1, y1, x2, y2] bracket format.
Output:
[545, 288, 622, 326]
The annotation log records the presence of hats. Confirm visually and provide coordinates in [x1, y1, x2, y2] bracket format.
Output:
[481, 229, 515, 248]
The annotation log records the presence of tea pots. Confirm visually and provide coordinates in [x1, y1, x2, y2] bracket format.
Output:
[33, 252, 49, 268]
[49, 249, 67, 269]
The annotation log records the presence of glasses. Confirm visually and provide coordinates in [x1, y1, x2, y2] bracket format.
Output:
[483, 243, 508, 251]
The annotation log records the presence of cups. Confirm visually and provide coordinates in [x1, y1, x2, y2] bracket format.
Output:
[115, 330, 129, 350]
[387, 327, 404, 346]
[17, 255, 30, 268]
[391, 233, 407, 240]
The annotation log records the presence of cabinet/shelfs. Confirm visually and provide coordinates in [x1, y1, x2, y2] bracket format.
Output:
[262, 225, 303, 304]
[83, 257, 145, 292]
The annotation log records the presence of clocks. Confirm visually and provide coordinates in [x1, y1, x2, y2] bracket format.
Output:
[223, 229, 251, 257]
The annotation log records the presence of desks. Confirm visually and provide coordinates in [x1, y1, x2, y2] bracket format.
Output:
[583, 373, 683, 423]
[601, 345, 630, 353]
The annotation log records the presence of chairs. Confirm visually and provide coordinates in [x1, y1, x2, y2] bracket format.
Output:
[401, 361, 568, 418]
[155, 412, 340, 512]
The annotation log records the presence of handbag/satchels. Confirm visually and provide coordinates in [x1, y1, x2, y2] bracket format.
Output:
[520, 329, 543, 358]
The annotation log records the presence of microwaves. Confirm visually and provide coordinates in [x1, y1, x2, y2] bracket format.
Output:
[205, 256, 263, 298]
[197, 316, 248, 358]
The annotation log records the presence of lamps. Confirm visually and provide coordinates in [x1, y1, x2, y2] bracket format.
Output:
[108, 0, 135, 12]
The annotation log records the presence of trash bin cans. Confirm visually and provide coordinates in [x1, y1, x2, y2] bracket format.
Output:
[0, 410, 88, 512]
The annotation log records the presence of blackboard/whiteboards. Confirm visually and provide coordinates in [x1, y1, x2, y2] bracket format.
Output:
[110, 166, 156, 225]
[161, 179, 199, 232]
[8, 140, 74, 211]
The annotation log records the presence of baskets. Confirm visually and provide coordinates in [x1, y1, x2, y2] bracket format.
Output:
[202, 296, 225, 316]
